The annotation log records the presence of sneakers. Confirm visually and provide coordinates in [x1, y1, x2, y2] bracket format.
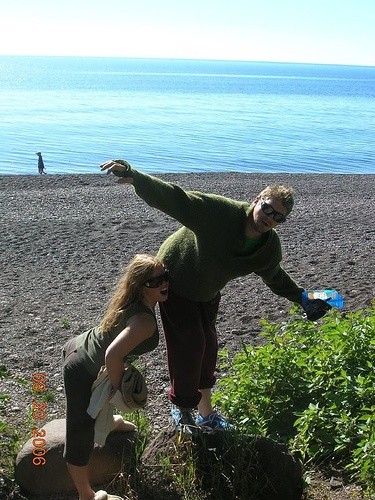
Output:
[171, 409, 199, 436]
[196, 414, 237, 432]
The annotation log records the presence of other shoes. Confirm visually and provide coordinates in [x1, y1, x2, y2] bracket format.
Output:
[101, 415, 136, 432]
[92, 490, 123, 500]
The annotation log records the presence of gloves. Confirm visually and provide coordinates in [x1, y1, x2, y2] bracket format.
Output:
[304, 299, 335, 321]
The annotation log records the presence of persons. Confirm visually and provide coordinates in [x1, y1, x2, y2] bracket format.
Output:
[100, 159, 333, 434]
[62, 254, 170, 500]
[36, 152, 47, 175]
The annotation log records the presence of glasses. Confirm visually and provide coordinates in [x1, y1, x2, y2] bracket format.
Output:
[142, 270, 170, 288]
[260, 197, 287, 224]
